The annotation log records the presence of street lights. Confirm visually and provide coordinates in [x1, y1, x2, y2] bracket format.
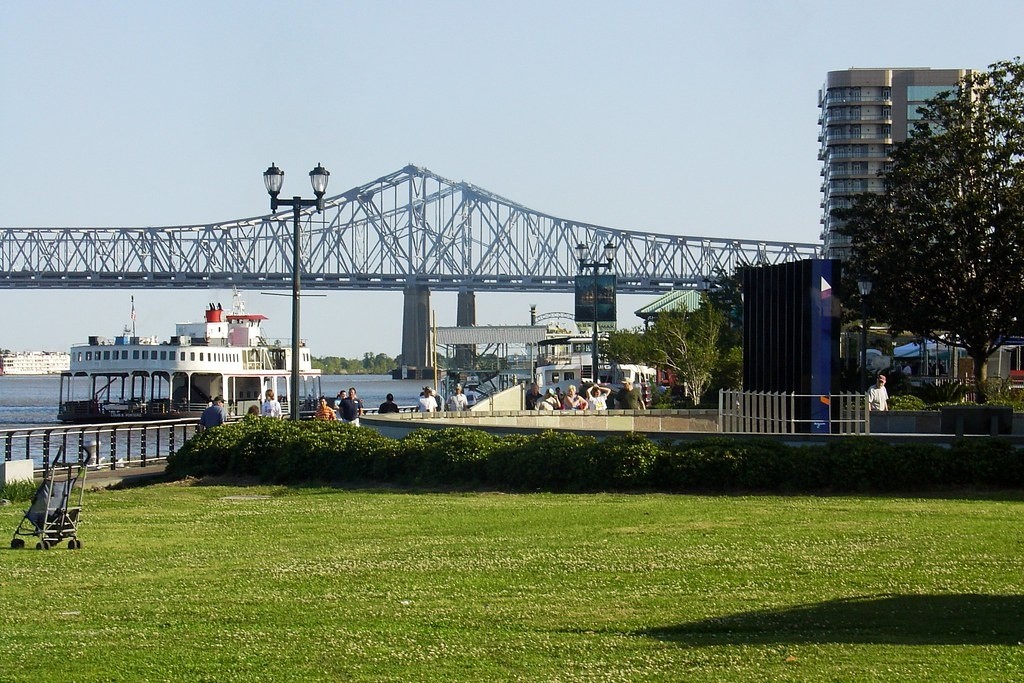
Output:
[262, 161, 330, 421]
[575, 240, 617, 384]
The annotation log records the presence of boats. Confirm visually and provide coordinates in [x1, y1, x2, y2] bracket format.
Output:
[57, 289, 323, 424]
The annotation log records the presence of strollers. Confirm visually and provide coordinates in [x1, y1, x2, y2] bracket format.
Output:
[12, 445, 91, 549]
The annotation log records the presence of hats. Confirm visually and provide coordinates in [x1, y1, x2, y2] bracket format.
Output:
[545, 388, 555, 397]
[621, 376, 632, 384]
[214, 396, 225, 402]
[878, 374, 887, 383]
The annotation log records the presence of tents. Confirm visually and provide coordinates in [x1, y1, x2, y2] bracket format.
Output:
[892, 333, 1024, 378]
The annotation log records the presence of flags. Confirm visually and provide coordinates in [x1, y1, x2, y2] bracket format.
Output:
[131, 302, 137, 320]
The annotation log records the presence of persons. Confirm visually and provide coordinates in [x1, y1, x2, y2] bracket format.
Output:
[104, 396, 187, 416]
[901, 360, 912, 377]
[925, 362, 934, 374]
[378, 393, 399, 414]
[414, 381, 468, 412]
[198, 388, 283, 433]
[933, 359, 945, 373]
[315, 387, 363, 428]
[526, 375, 666, 410]
[865, 375, 889, 411]
